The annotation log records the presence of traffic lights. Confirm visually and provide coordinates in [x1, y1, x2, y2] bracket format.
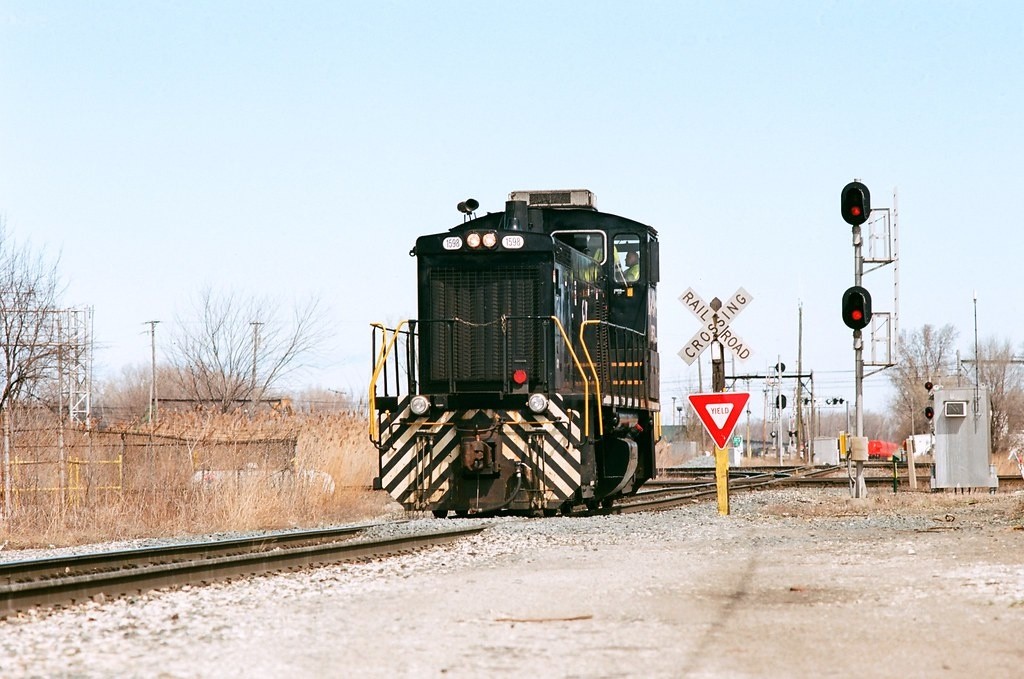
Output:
[925, 382, 933, 389]
[925, 407, 934, 419]
[842, 182, 871, 225]
[842, 286, 872, 329]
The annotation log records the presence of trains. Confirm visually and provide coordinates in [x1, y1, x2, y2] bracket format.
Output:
[867, 440, 898, 463]
[369, 189, 661, 520]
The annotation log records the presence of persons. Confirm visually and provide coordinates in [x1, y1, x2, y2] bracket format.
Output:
[619, 251, 641, 281]
